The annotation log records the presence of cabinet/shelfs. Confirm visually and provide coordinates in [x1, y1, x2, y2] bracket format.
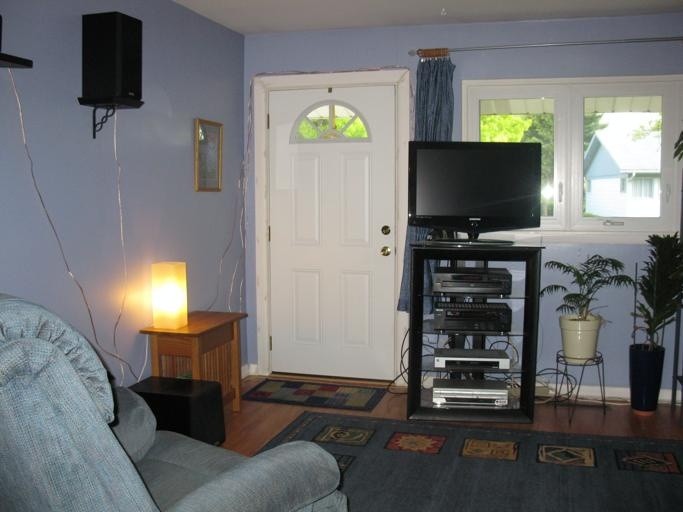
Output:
[406, 243, 546, 425]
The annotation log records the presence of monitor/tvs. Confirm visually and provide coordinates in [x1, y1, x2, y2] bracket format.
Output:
[407, 140, 542, 248]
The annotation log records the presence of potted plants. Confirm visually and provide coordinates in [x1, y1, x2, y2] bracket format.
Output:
[538, 253, 637, 365]
[628, 228, 682, 412]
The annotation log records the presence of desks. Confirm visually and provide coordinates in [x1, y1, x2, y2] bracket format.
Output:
[140, 309, 248, 413]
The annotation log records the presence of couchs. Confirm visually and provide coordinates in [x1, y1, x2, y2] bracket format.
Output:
[0, 294, 348, 512]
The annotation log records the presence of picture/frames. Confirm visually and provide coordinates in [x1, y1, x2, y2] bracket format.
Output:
[193, 115, 225, 195]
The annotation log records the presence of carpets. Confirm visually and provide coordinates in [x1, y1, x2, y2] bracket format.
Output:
[241, 376, 388, 413]
[252, 409, 683, 512]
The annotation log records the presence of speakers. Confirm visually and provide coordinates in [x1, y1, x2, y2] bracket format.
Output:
[81, 11, 145, 111]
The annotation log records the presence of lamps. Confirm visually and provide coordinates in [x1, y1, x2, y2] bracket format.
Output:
[151, 259, 188, 331]
